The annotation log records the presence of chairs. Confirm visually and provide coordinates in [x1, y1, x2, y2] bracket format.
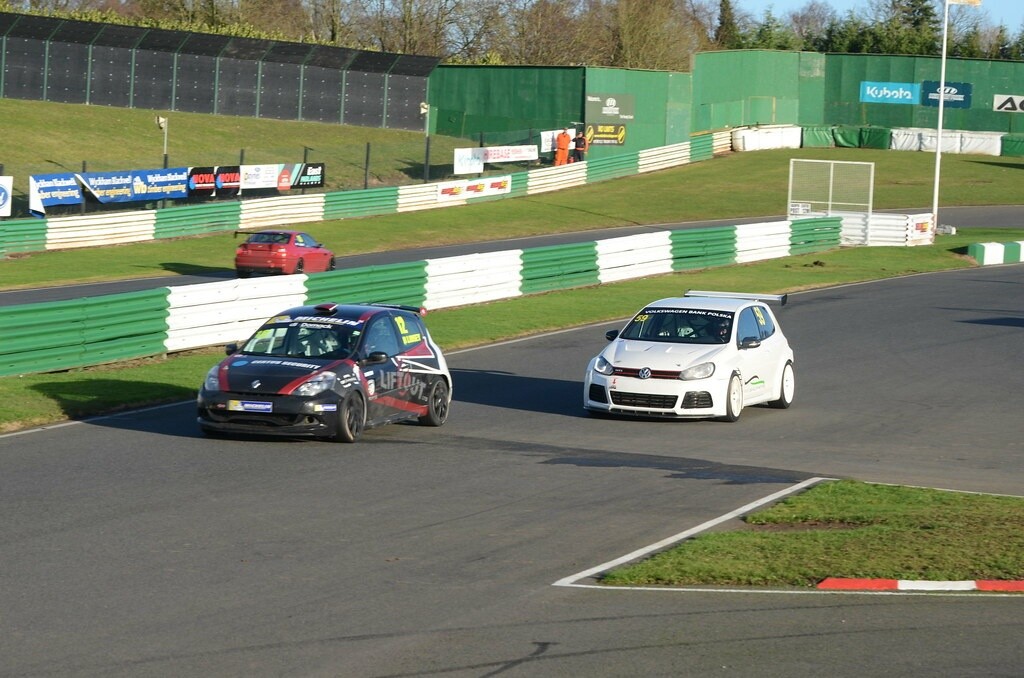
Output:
[657, 315, 697, 339]
[305, 329, 334, 357]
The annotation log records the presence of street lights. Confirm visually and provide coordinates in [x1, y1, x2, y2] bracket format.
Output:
[419, 101, 431, 184]
[154, 116, 169, 210]
[931, 1, 983, 237]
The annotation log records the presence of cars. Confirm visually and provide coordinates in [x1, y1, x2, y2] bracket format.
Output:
[195, 302, 453, 447]
[233, 229, 337, 276]
[583, 289, 796, 424]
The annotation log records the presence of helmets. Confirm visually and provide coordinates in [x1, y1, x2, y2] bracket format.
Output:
[713, 317, 731, 340]
[345, 327, 368, 354]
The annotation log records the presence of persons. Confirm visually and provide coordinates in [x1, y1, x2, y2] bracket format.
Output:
[572, 131, 588, 162]
[553, 128, 570, 166]
